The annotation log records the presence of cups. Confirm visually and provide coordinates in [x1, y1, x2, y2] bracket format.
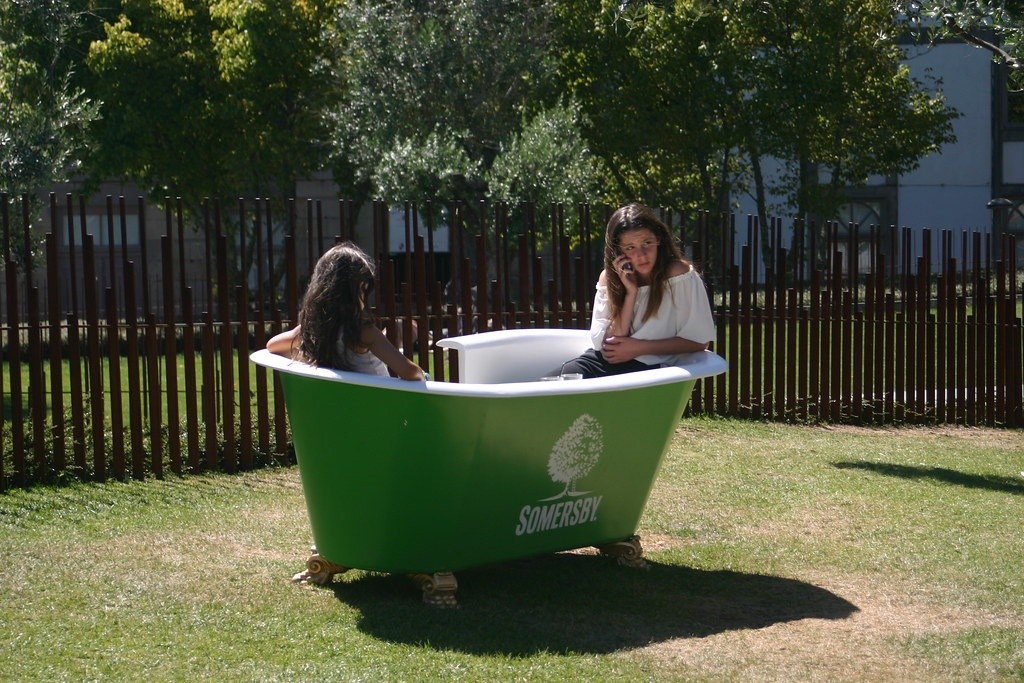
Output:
[539, 373, 583, 381]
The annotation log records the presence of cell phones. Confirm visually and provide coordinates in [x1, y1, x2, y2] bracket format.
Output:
[615, 247, 631, 270]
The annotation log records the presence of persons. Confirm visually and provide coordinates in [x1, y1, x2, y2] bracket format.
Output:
[266, 242, 430, 382]
[560, 201, 715, 380]
